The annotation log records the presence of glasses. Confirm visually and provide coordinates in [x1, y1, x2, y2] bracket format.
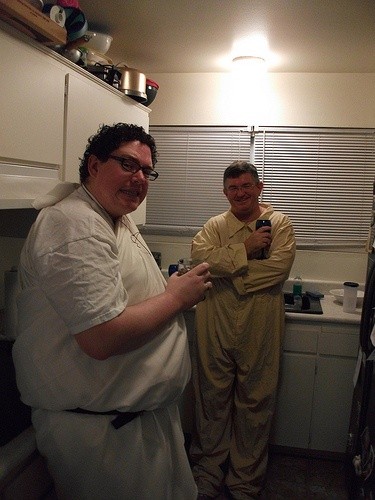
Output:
[225, 181, 259, 192]
[106, 153, 159, 181]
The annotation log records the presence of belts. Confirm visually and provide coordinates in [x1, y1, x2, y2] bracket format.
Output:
[64, 407, 147, 430]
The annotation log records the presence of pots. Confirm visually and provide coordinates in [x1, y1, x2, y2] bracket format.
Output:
[117, 67, 147, 104]
[47, 35, 93, 64]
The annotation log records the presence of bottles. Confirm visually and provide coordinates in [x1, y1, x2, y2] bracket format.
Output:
[293, 275, 303, 306]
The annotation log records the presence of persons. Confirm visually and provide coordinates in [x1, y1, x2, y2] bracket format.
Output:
[10, 122, 213, 500]
[192, 160, 295, 500]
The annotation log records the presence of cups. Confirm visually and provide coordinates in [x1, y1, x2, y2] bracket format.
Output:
[256, 219, 271, 259]
[343, 281, 359, 313]
[178, 258, 204, 310]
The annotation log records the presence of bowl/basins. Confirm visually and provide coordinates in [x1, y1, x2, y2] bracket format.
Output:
[329, 288, 364, 305]
[142, 78, 159, 107]
[85, 31, 113, 54]
